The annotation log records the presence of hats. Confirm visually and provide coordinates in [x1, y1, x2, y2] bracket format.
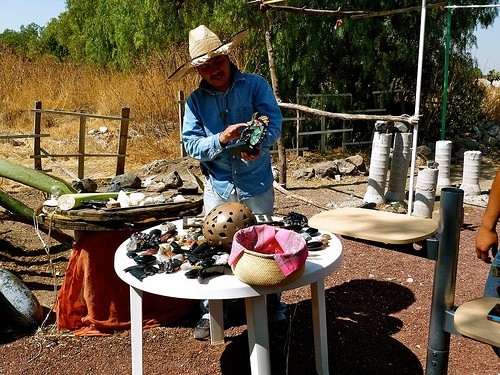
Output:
[168, 25, 251, 81]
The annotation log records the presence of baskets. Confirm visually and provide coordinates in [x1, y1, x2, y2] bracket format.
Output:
[228, 225, 308, 286]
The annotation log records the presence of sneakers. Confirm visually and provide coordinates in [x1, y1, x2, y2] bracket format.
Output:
[194, 315, 227, 339]
[268, 319, 294, 339]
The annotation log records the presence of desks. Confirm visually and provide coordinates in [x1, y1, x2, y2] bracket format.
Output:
[113, 213, 342, 375]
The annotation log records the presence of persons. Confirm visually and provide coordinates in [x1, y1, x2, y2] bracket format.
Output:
[475, 167, 500, 300]
[168, 23, 293, 338]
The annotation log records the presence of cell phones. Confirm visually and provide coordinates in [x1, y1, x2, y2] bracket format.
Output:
[487, 303, 500, 322]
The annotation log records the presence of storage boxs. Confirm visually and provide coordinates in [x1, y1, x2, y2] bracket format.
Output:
[229, 221, 309, 288]
